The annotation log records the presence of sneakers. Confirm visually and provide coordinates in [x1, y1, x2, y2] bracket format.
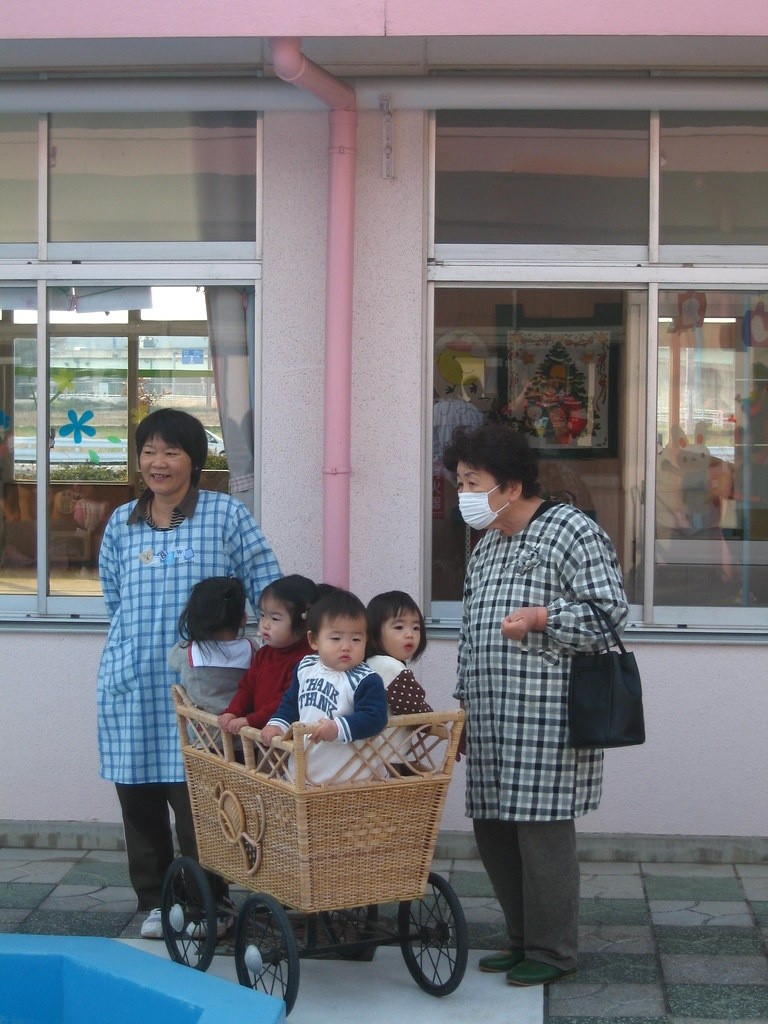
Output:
[141, 907, 165, 937]
[186, 914, 232, 938]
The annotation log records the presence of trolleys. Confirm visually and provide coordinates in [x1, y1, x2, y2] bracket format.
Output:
[160, 684, 471, 1018]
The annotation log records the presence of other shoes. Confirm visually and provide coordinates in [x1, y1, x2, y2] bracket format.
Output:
[478, 947, 525, 971]
[506, 960, 577, 986]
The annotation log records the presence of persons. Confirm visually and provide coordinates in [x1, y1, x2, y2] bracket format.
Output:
[1, 432, 110, 570]
[168, 575, 426, 788]
[451, 423, 629, 986]
[656, 361, 767, 605]
[99, 409, 287, 936]
[433, 354, 589, 518]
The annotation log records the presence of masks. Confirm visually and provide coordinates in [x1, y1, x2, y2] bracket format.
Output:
[458, 480, 510, 531]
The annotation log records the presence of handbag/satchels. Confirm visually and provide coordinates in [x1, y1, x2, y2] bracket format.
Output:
[567, 600, 647, 749]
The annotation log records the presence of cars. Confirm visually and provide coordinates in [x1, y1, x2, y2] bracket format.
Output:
[205, 429, 226, 456]
